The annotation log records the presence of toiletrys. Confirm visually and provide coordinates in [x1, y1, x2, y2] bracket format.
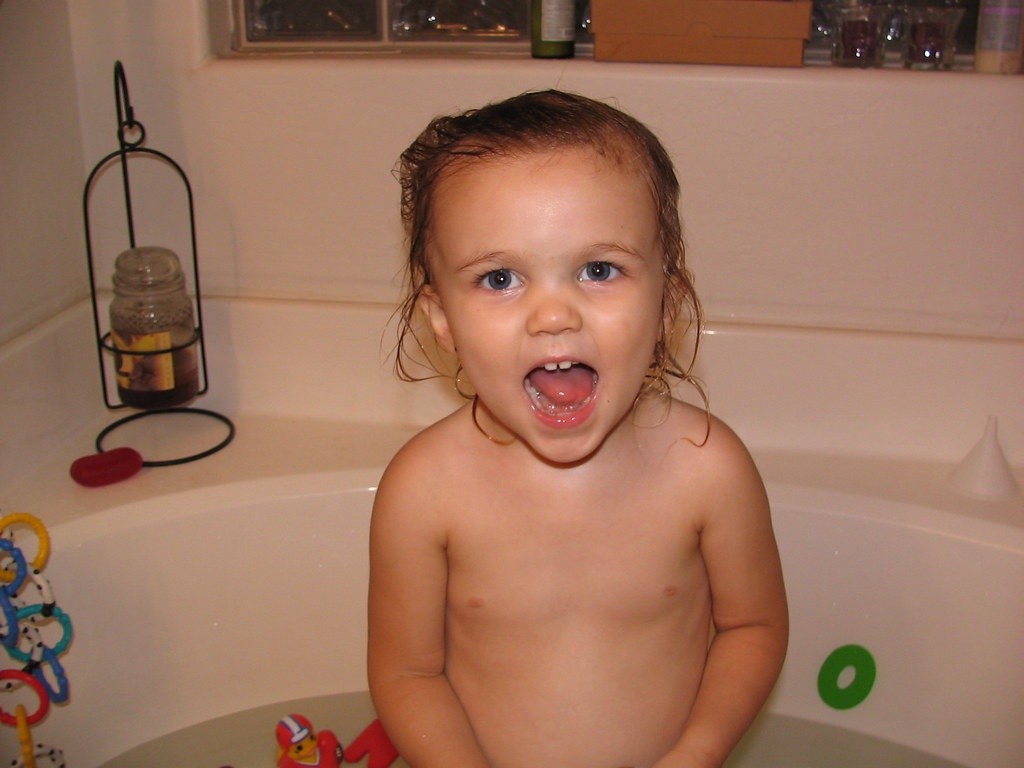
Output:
[973, 0, 1024, 74]
[529, 0, 577, 58]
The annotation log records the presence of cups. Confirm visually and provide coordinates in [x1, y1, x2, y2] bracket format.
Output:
[896, 0, 965, 70]
[818, 0, 898, 68]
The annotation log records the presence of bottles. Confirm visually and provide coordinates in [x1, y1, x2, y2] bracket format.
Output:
[530, 0, 577, 60]
[974, 0, 1024, 75]
[110, 246, 199, 409]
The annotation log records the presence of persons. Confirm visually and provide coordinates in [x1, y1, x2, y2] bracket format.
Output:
[364, 88, 790, 768]
[274, 714, 343, 768]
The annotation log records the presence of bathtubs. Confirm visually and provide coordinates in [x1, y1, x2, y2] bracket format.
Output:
[0, 291, 1024, 768]
